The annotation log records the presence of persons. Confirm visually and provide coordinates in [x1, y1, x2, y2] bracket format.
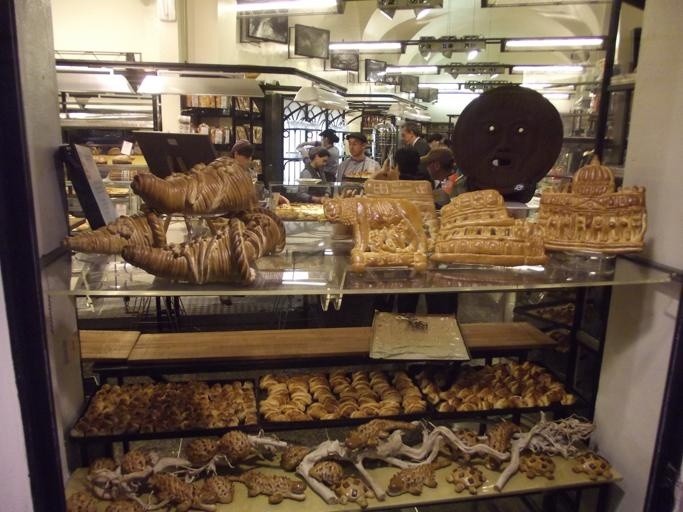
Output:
[392, 122, 468, 209]
[334, 132, 381, 199]
[231, 140, 268, 190]
[296, 146, 331, 203]
[297, 129, 339, 199]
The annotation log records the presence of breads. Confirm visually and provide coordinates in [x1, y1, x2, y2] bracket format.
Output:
[182, 435, 218, 467]
[145, 472, 216, 512]
[540, 154, 645, 252]
[66, 488, 98, 512]
[105, 498, 149, 512]
[232, 469, 307, 504]
[363, 178, 436, 214]
[386, 461, 450, 497]
[88, 458, 117, 474]
[206, 205, 287, 261]
[280, 443, 310, 471]
[121, 445, 157, 473]
[222, 430, 251, 464]
[345, 419, 417, 449]
[121, 220, 253, 289]
[431, 188, 517, 222]
[432, 223, 548, 266]
[447, 463, 487, 494]
[330, 477, 376, 508]
[323, 195, 428, 272]
[451, 428, 480, 462]
[200, 474, 234, 505]
[132, 156, 255, 217]
[70, 381, 257, 437]
[484, 419, 521, 471]
[309, 460, 344, 485]
[418, 359, 577, 416]
[62, 203, 166, 254]
[520, 452, 555, 480]
[258, 369, 430, 423]
[572, 450, 613, 480]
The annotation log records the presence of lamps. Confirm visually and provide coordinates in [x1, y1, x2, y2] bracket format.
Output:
[230, 0, 345, 17]
[325, 42, 408, 55]
[388, 64, 441, 76]
[509, 64, 588, 75]
[416, 34, 487, 61]
[415, 8, 431, 20]
[501, 37, 604, 51]
[378, 2, 396, 20]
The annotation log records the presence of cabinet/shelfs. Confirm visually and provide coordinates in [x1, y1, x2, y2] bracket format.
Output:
[2, 240, 683, 512]
[180, 75, 284, 190]
[563, 1, 644, 394]
[263, 94, 363, 184]
[58, 91, 162, 153]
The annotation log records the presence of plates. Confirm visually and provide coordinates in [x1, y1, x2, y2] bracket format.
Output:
[294, 178, 322, 185]
[342, 175, 367, 183]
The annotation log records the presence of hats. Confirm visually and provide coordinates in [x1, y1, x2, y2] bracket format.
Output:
[420, 148, 453, 163]
[319, 129, 336, 136]
[346, 133, 366, 142]
[308, 147, 325, 156]
[231, 140, 251, 153]
